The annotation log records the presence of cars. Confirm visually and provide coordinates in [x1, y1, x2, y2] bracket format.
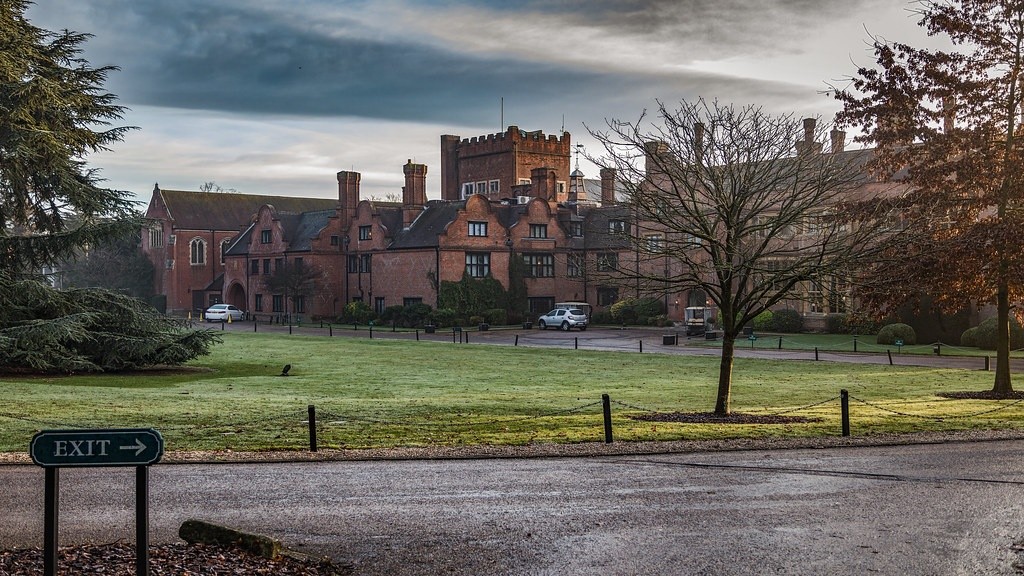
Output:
[205, 304, 245, 323]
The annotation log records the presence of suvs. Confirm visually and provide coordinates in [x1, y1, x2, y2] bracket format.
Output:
[538, 309, 588, 331]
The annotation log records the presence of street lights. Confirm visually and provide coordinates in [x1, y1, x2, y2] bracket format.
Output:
[209, 225, 216, 305]
[343, 234, 352, 305]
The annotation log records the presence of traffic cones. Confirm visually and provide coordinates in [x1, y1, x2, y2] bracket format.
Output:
[188, 312, 192, 321]
[227, 311, 232, 324]
[200, 312, 203, 322]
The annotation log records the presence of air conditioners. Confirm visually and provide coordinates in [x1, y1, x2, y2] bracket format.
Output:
[517, 196, 530, 205]
